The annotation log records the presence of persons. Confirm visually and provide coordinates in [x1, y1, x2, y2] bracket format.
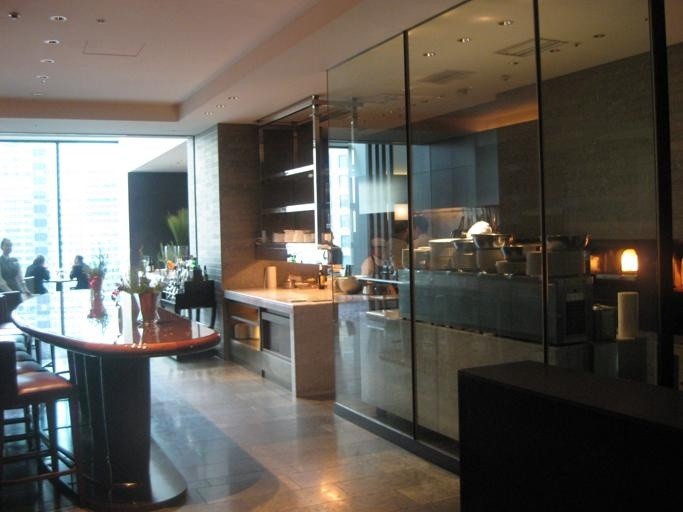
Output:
[0, 239, 31, 298]
[69, 256, 92, 289]
[321, 216, 436, 295]
[24, 255, 50, 294]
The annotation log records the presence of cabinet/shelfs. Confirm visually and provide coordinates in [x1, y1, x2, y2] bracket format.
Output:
[160, 278, 217, 361]
[223, 289, 334, 397]
[257, 94, 321, 248]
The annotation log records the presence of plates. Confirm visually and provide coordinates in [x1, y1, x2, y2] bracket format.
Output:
[401, 234, 460, 269]
[527, 250, 584, 277]
[271, 228, 331, 242]
[452, 251, 526, 274]
[471, 234, 511, 249]
[295, 283, 309, 288]
[233, 324, 260, 340]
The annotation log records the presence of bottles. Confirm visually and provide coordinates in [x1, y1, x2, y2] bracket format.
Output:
[316, 262, 325, 288]
[145, 256, 155, 273]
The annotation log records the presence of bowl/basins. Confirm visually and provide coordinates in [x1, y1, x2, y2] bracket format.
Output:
[452, 241, 476, 251]
[335, 275, 367, 294]
[501, 245, 540, 261]
[540, 232, 592, 251]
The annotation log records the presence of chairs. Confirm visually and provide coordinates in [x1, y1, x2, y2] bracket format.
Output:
[0, 271, 102, 512]
